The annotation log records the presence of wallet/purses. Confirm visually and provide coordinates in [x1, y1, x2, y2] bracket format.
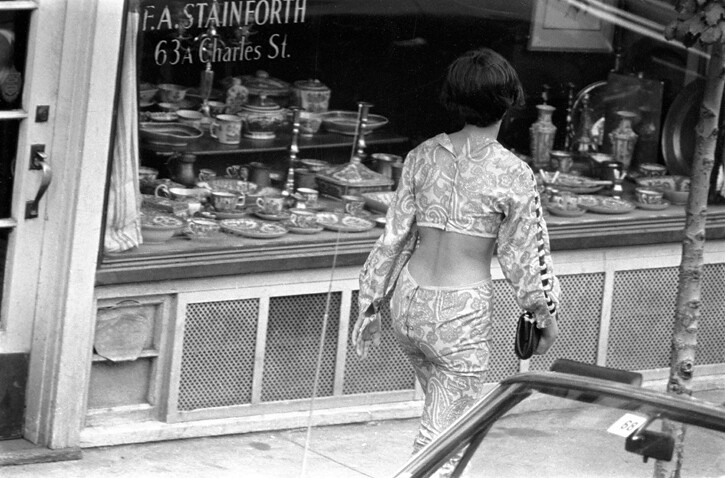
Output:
[515, 311, 541, 360]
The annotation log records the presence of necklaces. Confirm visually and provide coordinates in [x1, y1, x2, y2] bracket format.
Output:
[462, 129, 495, 140]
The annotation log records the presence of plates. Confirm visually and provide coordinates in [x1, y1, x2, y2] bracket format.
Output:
[139, 179, 395, 239]
[535, 170, 691, 218]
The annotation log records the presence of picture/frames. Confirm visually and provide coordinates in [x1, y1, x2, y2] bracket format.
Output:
[526, 0, 617, 54]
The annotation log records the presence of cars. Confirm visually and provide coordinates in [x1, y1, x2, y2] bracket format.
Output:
[383, 358, 725, 478]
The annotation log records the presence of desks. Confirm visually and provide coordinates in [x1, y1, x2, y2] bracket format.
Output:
[149, 130, 410, 157]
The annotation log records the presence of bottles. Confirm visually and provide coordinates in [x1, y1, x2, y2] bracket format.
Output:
[529, 103, 637, 166]
[288, 81, 331, 114]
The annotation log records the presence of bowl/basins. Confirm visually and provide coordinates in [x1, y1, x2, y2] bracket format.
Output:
[318, 109, 388, 135]
[137, 71, 222, 147]
[515, 149, 669, 206]
[289, 111, 322, 137]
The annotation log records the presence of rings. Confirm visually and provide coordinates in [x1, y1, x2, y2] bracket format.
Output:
[364, 339, 369, 341]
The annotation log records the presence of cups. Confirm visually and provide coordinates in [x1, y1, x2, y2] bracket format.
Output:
[176, 101, 242, 144]
[188, 218, 220, 237]
[134, 154, 404, 227]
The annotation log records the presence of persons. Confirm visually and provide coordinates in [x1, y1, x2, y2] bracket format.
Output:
[351, 50, 561, 478]
